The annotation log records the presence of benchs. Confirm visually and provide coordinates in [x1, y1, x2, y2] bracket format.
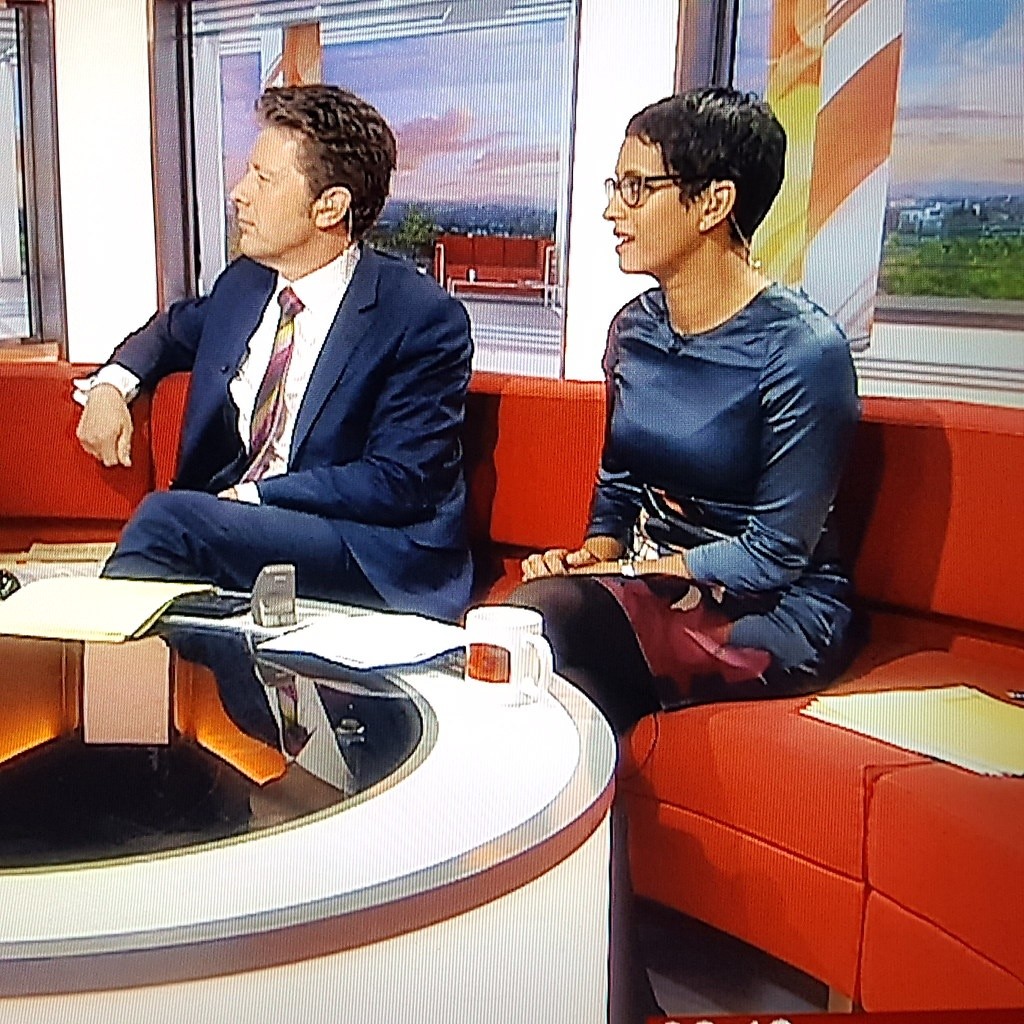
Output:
[436, 229, 559, 308]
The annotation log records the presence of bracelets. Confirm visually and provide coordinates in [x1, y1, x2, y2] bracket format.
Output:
[617, 558, 636, 576]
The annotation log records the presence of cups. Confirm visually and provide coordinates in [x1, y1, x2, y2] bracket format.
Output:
[466, 606, 553, 705]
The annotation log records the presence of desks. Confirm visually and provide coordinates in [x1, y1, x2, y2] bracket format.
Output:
[0, 573, 620, 1024]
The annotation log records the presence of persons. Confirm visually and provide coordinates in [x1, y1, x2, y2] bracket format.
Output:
[503, 82, 862, 1024]
[74, 83, 475, 626]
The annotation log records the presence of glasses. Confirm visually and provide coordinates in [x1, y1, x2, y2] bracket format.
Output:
[603, 173, 683, 208]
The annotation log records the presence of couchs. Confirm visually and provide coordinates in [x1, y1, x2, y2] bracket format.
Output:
[0, 361, 1024, 1014]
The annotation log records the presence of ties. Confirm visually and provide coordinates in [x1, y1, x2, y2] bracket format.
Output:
[236, 286, 305, 483]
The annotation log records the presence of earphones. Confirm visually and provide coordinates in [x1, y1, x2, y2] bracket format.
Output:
[316, 199, 333, 214]
[707, 197, 720, 214]
